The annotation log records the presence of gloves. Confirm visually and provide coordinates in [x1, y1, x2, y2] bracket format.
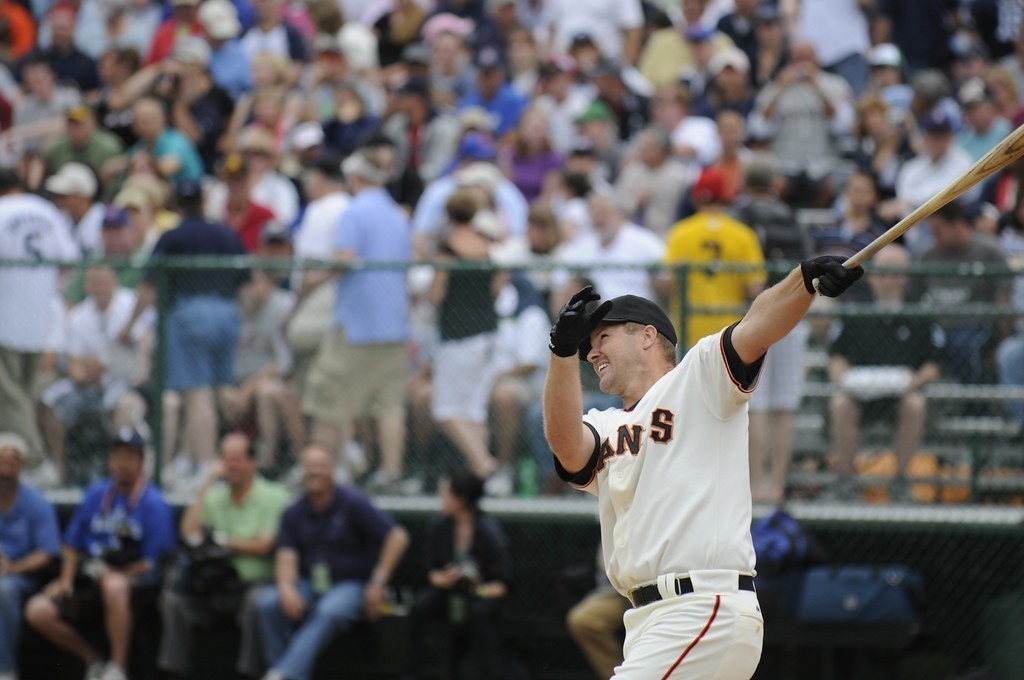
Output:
[801, 255, 864, 298]
[549, 285, 613, 358]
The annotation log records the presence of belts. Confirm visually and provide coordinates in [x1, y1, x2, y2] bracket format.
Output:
[630, 575, 755, 608]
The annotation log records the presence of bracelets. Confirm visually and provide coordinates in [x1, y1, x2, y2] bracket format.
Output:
[479, 583, 491, 597]
[369, 572, 386, 587]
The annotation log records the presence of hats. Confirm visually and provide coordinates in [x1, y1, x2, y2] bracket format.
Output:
[930, 97, 962, 129]
[340, 148, 393, 187]
[114, 187, 147, 214]
[45, 162, 99, 201]
[579, 295, 677, 360]
[106, 429, 141, 457]
[457, 129, 499, 161]
[691, 169, 734, 202]
[866, 42, 900, 66]
[104, 209, 126, 227]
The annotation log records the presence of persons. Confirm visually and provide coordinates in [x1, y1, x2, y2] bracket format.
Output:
[544, 256, 866, 680]
[0, 0, 1024, 679]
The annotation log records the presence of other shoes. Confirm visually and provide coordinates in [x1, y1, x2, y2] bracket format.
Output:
[84, 658, 128, 680]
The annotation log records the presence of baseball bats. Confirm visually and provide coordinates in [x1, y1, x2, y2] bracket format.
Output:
[813, 123, 1024, 296]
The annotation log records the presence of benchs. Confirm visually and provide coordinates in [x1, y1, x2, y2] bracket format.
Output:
[798, 378, 1024, 506]
